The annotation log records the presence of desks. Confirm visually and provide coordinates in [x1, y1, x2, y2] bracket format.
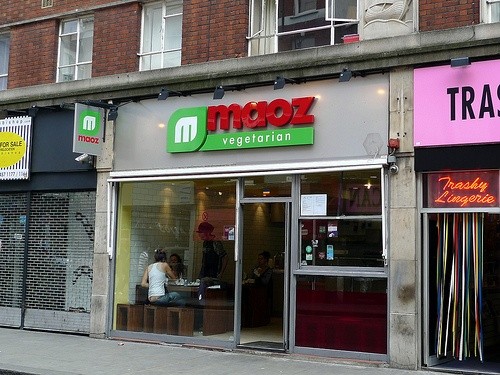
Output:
[135, 284, 200, 304]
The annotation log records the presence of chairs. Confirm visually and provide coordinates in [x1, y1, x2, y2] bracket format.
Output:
[245, 279, 273, 328]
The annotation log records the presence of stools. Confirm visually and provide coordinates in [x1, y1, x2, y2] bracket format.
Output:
[116, 304, 195, 337]
[203, 299, 234, 335]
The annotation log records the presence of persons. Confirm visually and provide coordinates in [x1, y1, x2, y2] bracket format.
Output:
[170, 254, 184, 277]
[141, 249, 187, 303]
[196, 222, 228, 330]
[251, 251, 272, 282]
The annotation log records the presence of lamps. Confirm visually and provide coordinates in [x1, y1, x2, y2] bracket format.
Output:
[338, 68, 366, 83]
[262, 176, 271, 194]
[108, 98, 139, 121]
[451, 57, 472, 68]
[213, 85, 245, 99]
[274, 76, 306, 90]
[158, 88, 191, 100]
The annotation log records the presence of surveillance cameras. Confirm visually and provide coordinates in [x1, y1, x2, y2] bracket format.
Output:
[390, 164, 399, 174]
[75, 153, 89, 161]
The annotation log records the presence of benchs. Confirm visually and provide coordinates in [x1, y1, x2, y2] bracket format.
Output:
[295, 288, 388, 354]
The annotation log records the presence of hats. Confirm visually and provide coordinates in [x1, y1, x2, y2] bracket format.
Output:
[196, 222, 214, 234]
